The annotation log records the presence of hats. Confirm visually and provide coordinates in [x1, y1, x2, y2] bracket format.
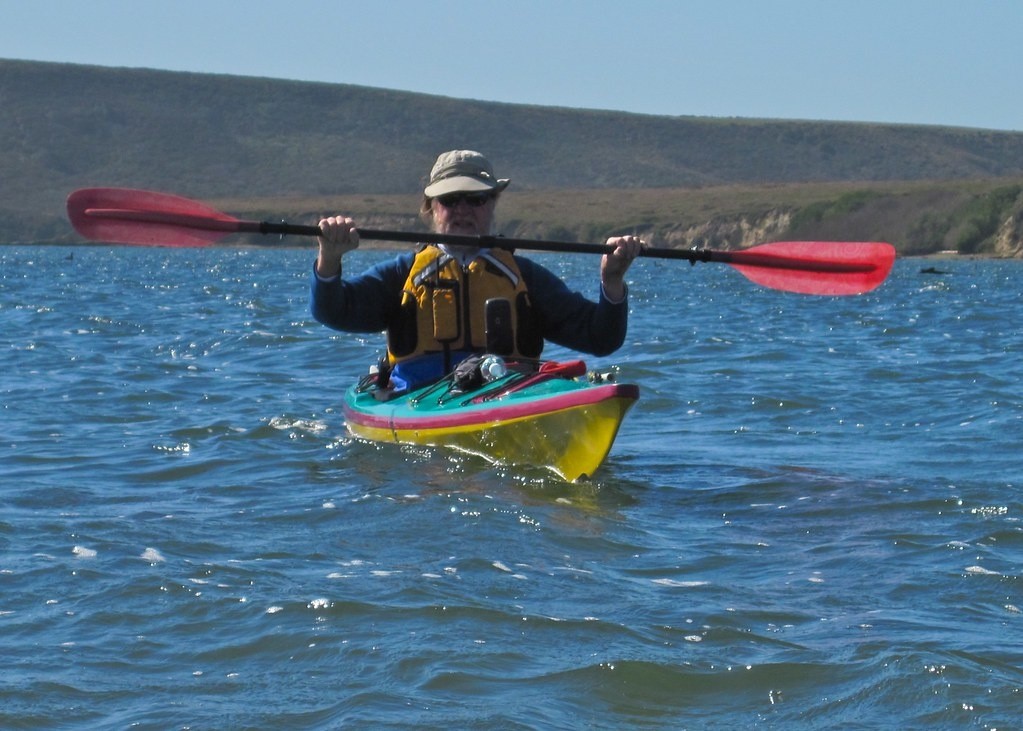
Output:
[423, 150, 510, 198]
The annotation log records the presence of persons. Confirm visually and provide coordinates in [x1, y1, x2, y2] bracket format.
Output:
[310, 150, 648, 390]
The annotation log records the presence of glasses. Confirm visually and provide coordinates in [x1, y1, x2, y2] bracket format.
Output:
[437, 192, 492, 208]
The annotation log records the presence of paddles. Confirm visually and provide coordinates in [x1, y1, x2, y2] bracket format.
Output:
[65, 187, 896, 296]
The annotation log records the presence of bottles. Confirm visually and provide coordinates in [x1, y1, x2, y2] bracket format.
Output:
[480, 357, 507, 382]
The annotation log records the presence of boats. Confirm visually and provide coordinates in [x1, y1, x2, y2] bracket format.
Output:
[346, 357, 640, 483]
